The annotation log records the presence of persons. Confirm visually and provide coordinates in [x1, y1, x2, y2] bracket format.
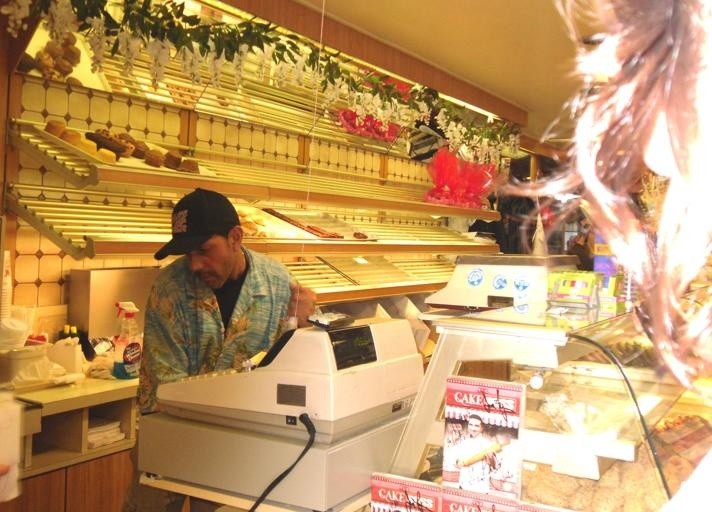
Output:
[486, 1, 712, 512]
[128, 186, 318, 512]
[450, 416, 501, 493]
[568, 217, 594, 271]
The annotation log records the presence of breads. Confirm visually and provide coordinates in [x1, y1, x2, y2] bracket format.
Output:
[145, 149, 164, 167]
[120, 133, 135, 143]
[44, 67, 65, 81]
[181, 159, 199, 173]
[111, 136, 135, 156]
[75, 140, 97, 156]
[164, 151, 181, 169]
[66, 77, 83, 86]
[36, 51, 53, 71]
[55, 55, 73, 77]
[45, 121, 65, 138]
[132, 140, 149, 158]
[95, 128, 117, 140]
[97, 148, 117, 164]
[59, 130, 81, 145]
[62, 32, 76, 46]
[64, 46, 81, 65]
[45, 41, 64, 58]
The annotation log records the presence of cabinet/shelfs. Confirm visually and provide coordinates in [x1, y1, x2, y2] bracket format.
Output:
[2, 117, 505, 304]
[18, 450, 136, 510]
[387, 307, 712, 512]
[316, 291, 440, 365]
[20, 374, 150, 482]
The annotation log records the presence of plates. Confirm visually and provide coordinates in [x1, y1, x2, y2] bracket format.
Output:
[34, 123, 217, 178]
[262, 208, 377, 240]
[234, 206, 322, 240]
[25, 19, 112, 93]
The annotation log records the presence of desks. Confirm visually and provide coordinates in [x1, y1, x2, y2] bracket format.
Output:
[139, 472, 373, 512]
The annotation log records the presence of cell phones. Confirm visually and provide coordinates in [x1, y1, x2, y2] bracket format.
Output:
[306, 311, 355, 328]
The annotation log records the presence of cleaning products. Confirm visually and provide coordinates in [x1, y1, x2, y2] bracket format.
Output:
[113, 302, 143, 378]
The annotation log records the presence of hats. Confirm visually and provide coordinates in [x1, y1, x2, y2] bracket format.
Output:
[154, 189, 240, 260]
